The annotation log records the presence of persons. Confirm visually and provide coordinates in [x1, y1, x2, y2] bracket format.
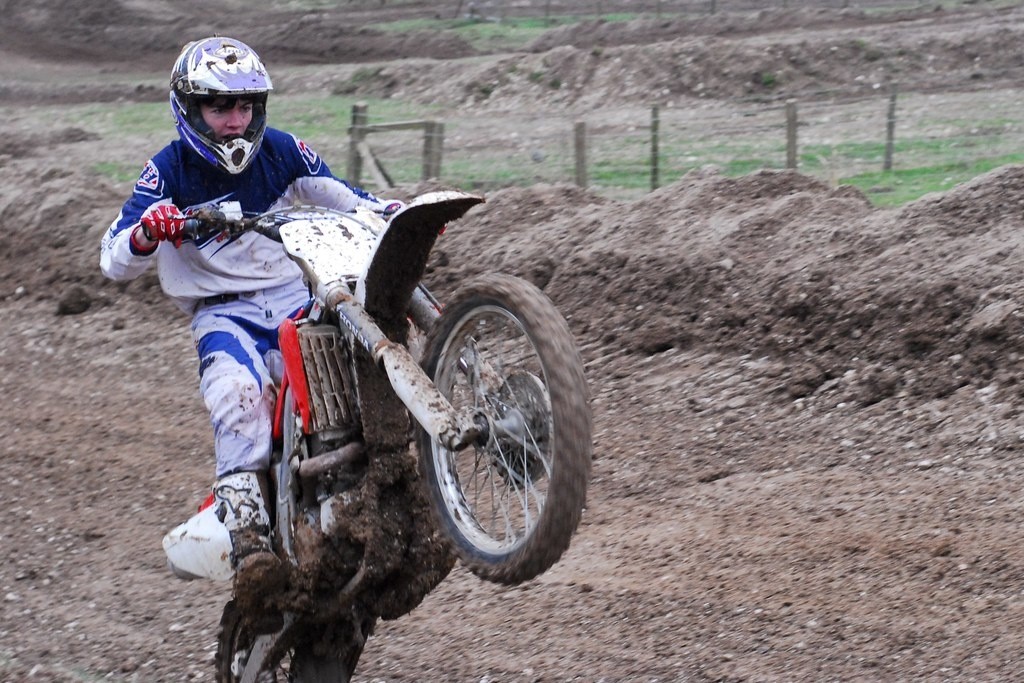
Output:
[100, 37, 447, 581]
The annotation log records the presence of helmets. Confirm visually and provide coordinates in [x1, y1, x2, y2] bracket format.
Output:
[169, 32, 273, 173]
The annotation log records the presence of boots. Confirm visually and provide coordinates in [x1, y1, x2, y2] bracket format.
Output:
[212, 470, 292, 599]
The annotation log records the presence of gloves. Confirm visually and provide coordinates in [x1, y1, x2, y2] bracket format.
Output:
[439, 223, 447, 235]
[141, 203, 186, 250]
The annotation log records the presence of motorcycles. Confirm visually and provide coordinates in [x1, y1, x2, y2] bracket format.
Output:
[141, 190, 598, 683]
